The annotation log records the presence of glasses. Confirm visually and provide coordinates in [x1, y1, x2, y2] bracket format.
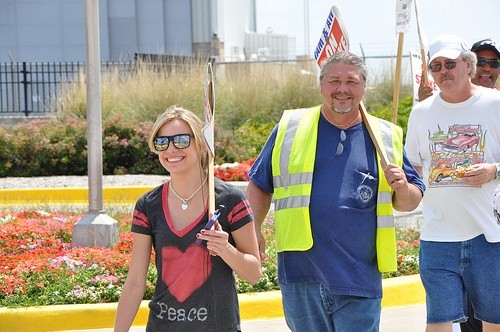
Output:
[476, 58, 500, 68]
[153, 134, 193, 152]
[430, 60, 462, 72]
[335, 130, 346, 157]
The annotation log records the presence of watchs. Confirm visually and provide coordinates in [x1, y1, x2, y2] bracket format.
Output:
[492, 163, 500, 180]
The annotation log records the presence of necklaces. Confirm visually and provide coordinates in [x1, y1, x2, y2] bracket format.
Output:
[168, 173, 207, 211]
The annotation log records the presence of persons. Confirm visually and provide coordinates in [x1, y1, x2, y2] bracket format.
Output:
[114, 106, 261, 332]
[242, 51, 426, 332]
[406, 37, 500, 332]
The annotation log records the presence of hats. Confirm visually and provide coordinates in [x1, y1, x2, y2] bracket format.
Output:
[427, 32, 469, 65]
[471, 39, 500, 57]
